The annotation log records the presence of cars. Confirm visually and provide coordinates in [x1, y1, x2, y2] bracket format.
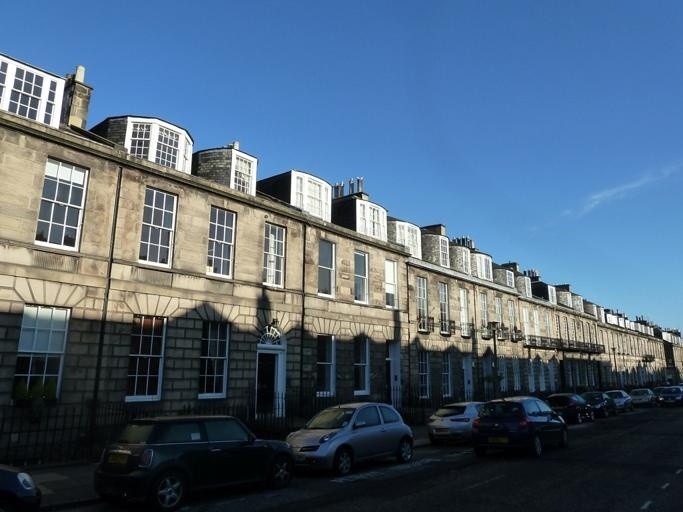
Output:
[0, 461, 43, 512]
[603, 389, 634, 413]
[653, 382, 683, 408]
[628, 388, 652, 408]
[285, 399, 415, 478]
[470, 393, 570, 459]
[91, 412, 295, 508]
[426, 400, 486, 447]
[545, 391, 595, 424]
[579, 390, 618, 418]
